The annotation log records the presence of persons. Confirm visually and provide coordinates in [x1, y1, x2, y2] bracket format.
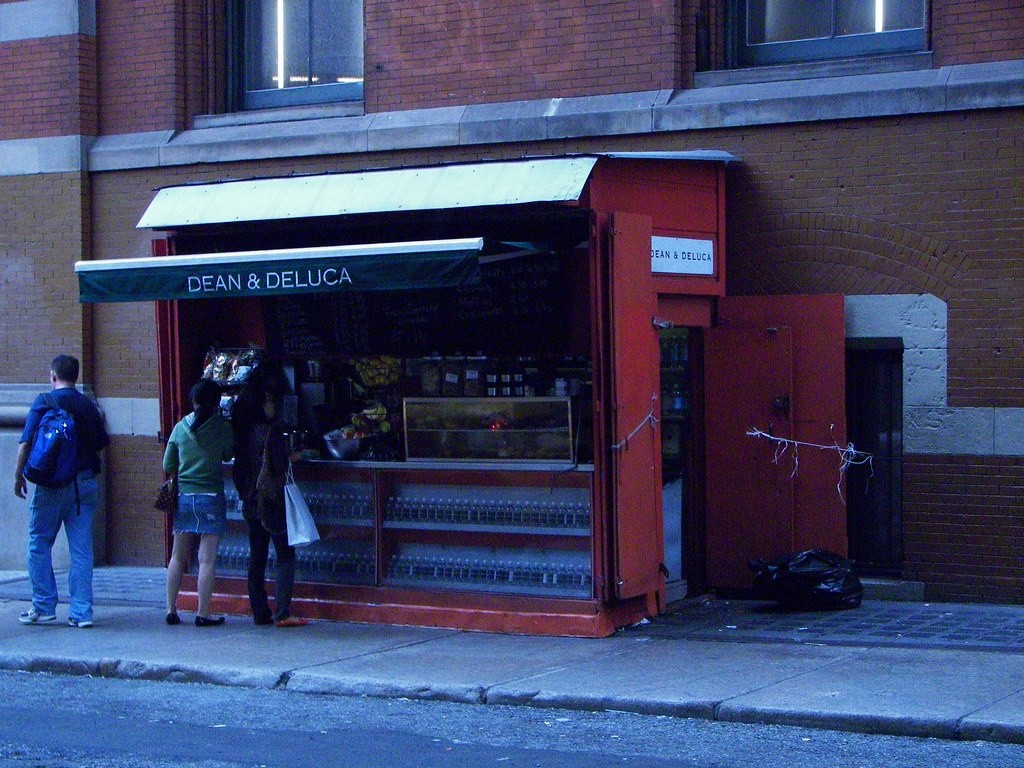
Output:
[386, 383, 414, 458]
[14, 355, 108, 627]
[230, 365, 309, 627]
[163, 382, 235, 625]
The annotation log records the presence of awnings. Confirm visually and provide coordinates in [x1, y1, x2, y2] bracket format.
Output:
[74, 238, 583, 304]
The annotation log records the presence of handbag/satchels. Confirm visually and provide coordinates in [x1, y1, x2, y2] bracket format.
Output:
[154, 471, 178, 514]
[284, 459, 321, 548]
[259, 478, 288, 535]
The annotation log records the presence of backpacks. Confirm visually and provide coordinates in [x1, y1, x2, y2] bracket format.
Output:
[24, 391, 78, 490]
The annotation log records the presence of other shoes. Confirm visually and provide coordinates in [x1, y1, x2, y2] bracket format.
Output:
[275, 618, 309, 627]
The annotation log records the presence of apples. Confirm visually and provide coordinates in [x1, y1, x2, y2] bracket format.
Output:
[342, 427, 369, 439]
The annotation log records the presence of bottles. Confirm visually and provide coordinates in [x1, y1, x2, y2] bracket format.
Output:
[290, 551, 376, 586]
[659, 382, 689, 412]
[382, 495, 592, 528]
[264, 548, 277, 578]
[386, 554, 592, 591]
[194, 543, 250, 575]
[222, 490, 243, 513]
[657, 336, 688, 367]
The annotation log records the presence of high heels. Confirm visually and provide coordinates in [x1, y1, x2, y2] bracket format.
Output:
[165, 613, 181, 625]
[194, 615, 225, 627]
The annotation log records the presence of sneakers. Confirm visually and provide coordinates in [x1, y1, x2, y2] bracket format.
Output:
[67, 616, 93, 628]
[18, 607, 57, 623]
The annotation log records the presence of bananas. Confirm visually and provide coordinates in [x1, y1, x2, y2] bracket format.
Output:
[363, 401, 387, 421]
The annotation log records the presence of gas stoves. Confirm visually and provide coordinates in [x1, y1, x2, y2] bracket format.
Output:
[297, 492, 375, 520]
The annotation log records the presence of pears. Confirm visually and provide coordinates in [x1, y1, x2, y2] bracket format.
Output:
[356, 357, 401, 386]
